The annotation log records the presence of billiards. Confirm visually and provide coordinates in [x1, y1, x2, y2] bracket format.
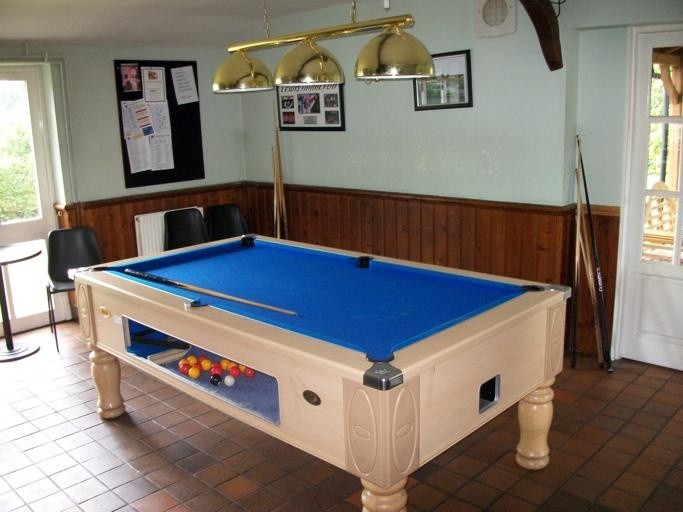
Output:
[179, 355, 255, 386]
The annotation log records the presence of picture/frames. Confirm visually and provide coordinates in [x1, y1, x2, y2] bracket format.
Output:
[413, 50, 472, 111]
[276, 85, 345, 131]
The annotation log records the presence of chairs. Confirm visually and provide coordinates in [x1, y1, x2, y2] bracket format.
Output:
[164, 209, 210, 250]
[46, 227, 104, 353]
[206, 203, 248, 241]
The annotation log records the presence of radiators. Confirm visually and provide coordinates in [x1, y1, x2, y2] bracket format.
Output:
[134, 206, 204, 256]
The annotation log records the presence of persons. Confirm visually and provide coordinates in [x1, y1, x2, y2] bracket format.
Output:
[122, 66, 140, 93]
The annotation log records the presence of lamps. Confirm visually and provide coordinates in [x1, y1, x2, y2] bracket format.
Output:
[212, 0, 435, 93]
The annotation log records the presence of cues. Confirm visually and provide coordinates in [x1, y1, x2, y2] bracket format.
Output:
[124, 267, 298, 317]
[569, 134, 614, 374]
[272, 101, 289, 241]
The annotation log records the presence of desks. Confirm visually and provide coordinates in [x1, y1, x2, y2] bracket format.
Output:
[0, 245, 42, 362]
[75, 233, 573, 512]
